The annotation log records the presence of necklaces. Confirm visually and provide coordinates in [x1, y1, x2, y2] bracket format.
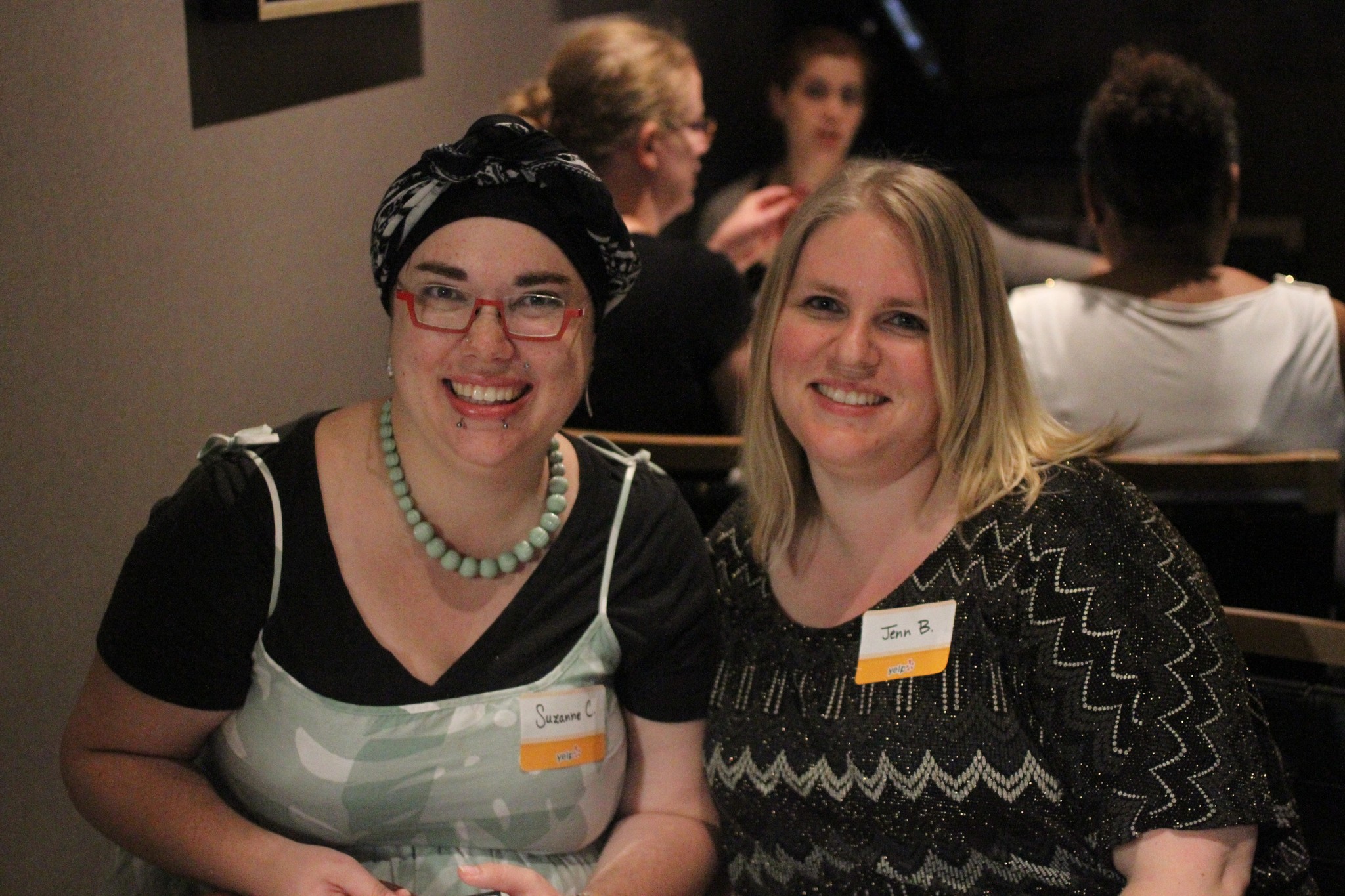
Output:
[381, 394, 570, 577]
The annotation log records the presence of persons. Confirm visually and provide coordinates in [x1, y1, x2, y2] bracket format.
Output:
[701, 35, 1111, 295]
[698, 162, 1322, 896]
[58, 113, 717, 896]
[495, 14, 802, 538]
[1003, 51, 1345, 458]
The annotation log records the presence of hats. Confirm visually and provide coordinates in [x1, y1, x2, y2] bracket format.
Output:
[368, 114, 638, 326]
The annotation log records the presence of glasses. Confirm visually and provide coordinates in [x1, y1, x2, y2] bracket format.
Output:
[392, 282, 593, 342]
[655, 118, 717, 143]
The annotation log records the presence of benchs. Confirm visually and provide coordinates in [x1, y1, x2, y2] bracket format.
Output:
[562, 429, 1345, 896]
